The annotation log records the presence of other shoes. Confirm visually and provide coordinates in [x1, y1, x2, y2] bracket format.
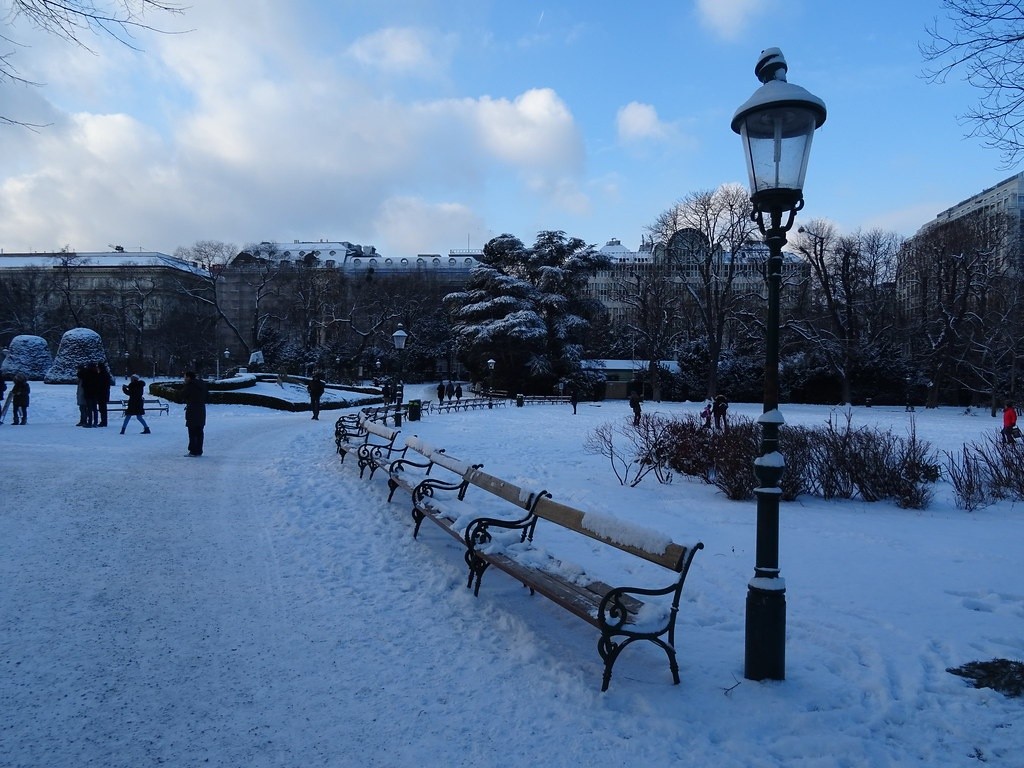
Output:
[312, 416, 319, 420]
[184, 452, 202, 457]
[97, 422, 107, 427]
[76, 422, 98, 428]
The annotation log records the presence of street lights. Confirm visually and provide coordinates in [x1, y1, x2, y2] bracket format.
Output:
[729, 47, 827, 678]
[392, 323, 408, 427]
[487, 359, 496, 409]
[224, 347, 230, 378]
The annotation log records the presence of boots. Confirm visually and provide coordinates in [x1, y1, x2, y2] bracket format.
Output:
[120, 428, 125, 434]
[11, 417, 19, 425]
[20, 416, 27, 425]
[140, 427, 150, 434]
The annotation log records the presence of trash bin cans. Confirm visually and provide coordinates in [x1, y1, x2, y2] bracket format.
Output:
[373, 377, 379, 387]
[865, 398, 872, 407]
[452, 371, 457, 382]
[465, 371, 470, 381]
[409, 400, 421, 421]
[438, 373, 442, 381]
[516, 394, 524, 407]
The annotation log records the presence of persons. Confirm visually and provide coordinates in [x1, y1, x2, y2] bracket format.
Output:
[705, 391, 728, 430]
[473, 379, 487, 397]
[452, 382, 462, 404]
[179, 370, 206, 457]
[1001, 399, 1018, 445]
[373, 379, 378, 387]
[0, 370, 7, 425]
[75, 360, 112, 428]
[569, 391, 577, 414]
[11, 373, 30, 425]
[445, 381, 454, 405]
[905, 391, 916, 412]
[382, 380, 397, 406]
[307, 374, 324, 420]
[437, 382, 445, 405]
[120, 375, 150, 434]
[630, 391, 644, 426]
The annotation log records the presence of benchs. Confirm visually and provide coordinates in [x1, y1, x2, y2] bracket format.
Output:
[334, 387, 703, 693]
[97, 399, 169, 417]
